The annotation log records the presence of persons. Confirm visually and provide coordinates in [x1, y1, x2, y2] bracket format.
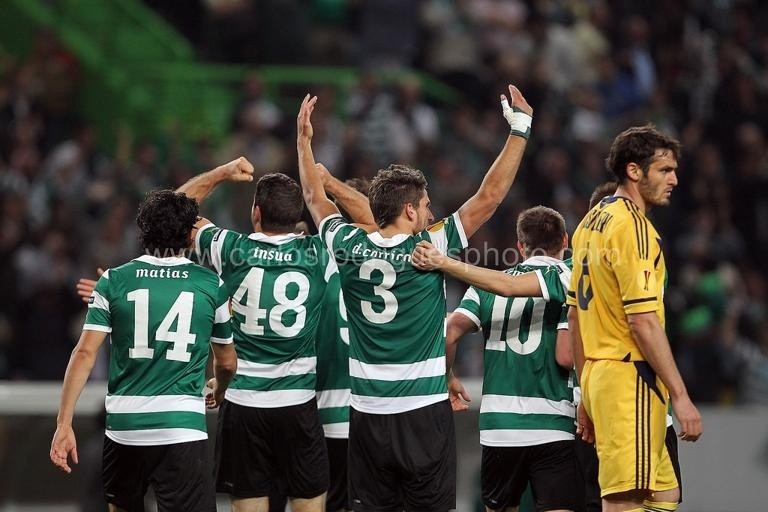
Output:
[294, 175, 372, 511]
[174, 155, 381, 512]
[50, 190, 238, 512]
[565, 126, 701, 512]
[415, 181, 682, 512]
[446, 202, 577, 512]
[295, 82, 536, 512]
[1, 2, 768, 408]
